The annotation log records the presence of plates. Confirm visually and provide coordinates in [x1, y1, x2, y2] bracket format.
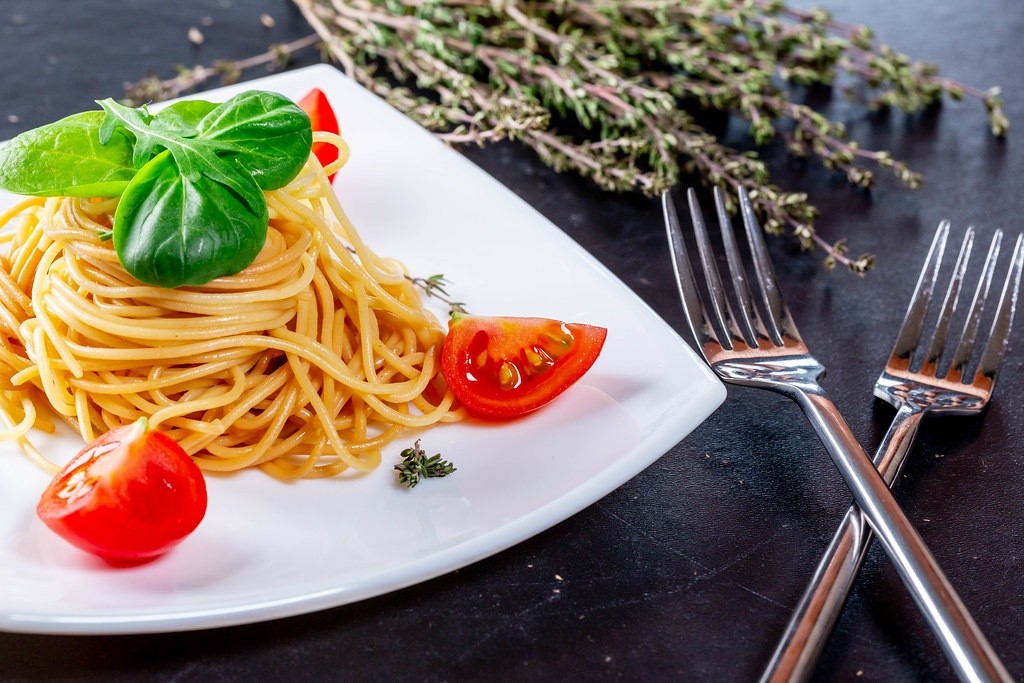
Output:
[0, 58, 732, 644]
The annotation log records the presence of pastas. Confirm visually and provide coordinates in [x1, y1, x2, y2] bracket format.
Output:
[0, 91, 471, 481]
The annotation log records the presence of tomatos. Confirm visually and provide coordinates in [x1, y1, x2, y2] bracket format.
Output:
[33, 419, 208, 568]
[443, 313, 613, 416]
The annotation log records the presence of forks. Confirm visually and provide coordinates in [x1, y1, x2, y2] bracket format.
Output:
[749, 214, 1024, 683]
[658, 185, 1016, 683]
